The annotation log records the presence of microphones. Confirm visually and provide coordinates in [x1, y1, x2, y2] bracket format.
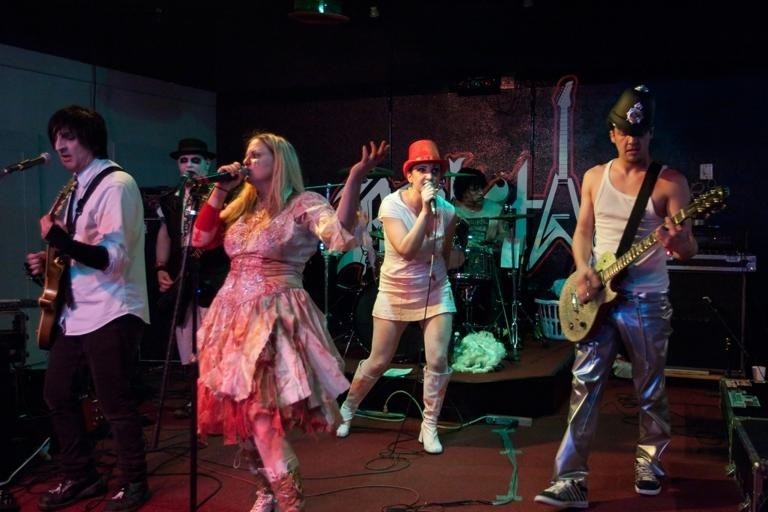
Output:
[195, 166, 247, 185]
[4, 152, 50, 172]
[424, 180, 436, 216]
[173, 171, 191, 199]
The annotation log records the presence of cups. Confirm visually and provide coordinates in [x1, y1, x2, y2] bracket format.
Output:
[751, 366, 766, 382]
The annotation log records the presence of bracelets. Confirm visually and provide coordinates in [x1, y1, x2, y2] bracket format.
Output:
[156, 264, 168, 274]
[215, 185, 229, 193]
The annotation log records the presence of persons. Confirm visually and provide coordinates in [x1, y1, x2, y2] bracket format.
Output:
[449, 167, 522, 350]
[154, 136, 244, 366]
[533, 83, 698, 510]
[190, 133, 392, 511]
[334, 138, 459, 456]
[23, 104, 152, 510]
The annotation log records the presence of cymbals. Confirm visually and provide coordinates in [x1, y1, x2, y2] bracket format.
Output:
[444, 172, 477, 178]
[466, 212, 536, 221]
[334, 166, 395, 178]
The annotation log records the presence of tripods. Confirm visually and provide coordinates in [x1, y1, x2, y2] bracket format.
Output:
[493, 218, 549, 361]
[322, 188, 370, 358]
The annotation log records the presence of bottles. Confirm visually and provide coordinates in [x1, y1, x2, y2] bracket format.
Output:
[533, 313, 542, 341]
[449, 332, 461, 364]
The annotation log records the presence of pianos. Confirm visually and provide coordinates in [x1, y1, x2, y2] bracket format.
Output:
[667, 255, 757, 274]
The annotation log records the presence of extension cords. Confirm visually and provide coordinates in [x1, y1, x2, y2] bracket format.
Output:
[364, 411, 404, 418]
[485, 415, 532, 427]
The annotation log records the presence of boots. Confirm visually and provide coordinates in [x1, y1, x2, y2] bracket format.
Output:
[256, 453, 306, 511]
[418, 365, 454, 454]
[245, 456, 278, 511]
[333, 358, 381, 437]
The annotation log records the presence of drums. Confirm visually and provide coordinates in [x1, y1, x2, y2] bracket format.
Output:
[447, 217, 469, 271]
[351, 279, 426, 363]
[450, 245, 495, 280]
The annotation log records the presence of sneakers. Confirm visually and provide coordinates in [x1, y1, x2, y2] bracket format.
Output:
[534, 476, 590, 508]
[632, 456, 663, 495]
[106, 478, 153, 512]
[37, 471, 108, 509]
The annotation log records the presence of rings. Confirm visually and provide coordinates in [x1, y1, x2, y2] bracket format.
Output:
[585, 288, 592, 298]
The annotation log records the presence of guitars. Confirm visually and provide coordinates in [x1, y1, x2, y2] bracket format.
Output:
[558, 184, 729, 344]
[149, 247, 206, 326]
[37, 179, 80, 350]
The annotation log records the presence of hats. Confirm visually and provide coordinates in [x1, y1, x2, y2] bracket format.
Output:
[606, 85, 656, 137]
[402, 140, 449, 179]
[169, 139, 216, 161]
